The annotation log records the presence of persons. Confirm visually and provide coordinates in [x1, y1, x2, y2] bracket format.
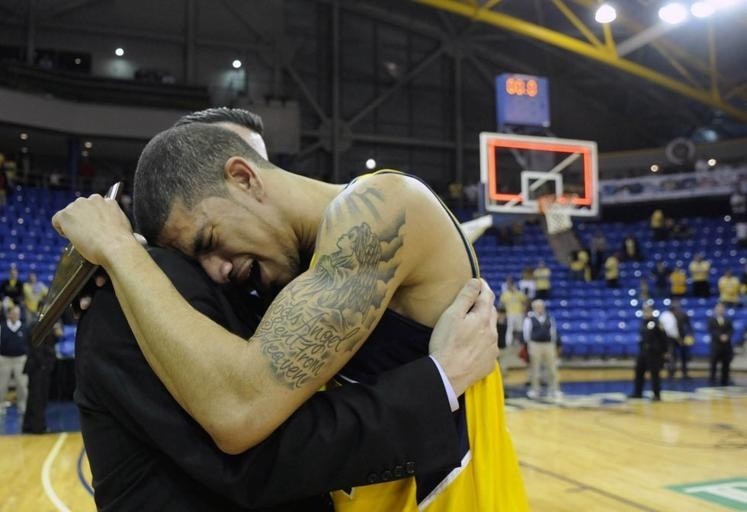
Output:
[45, 120, 531, 512]
[0, 267, 80, 432]
[495, 207, 746, 402]
[74, 104, 499, 512]
[0, 153, 10, 206]
